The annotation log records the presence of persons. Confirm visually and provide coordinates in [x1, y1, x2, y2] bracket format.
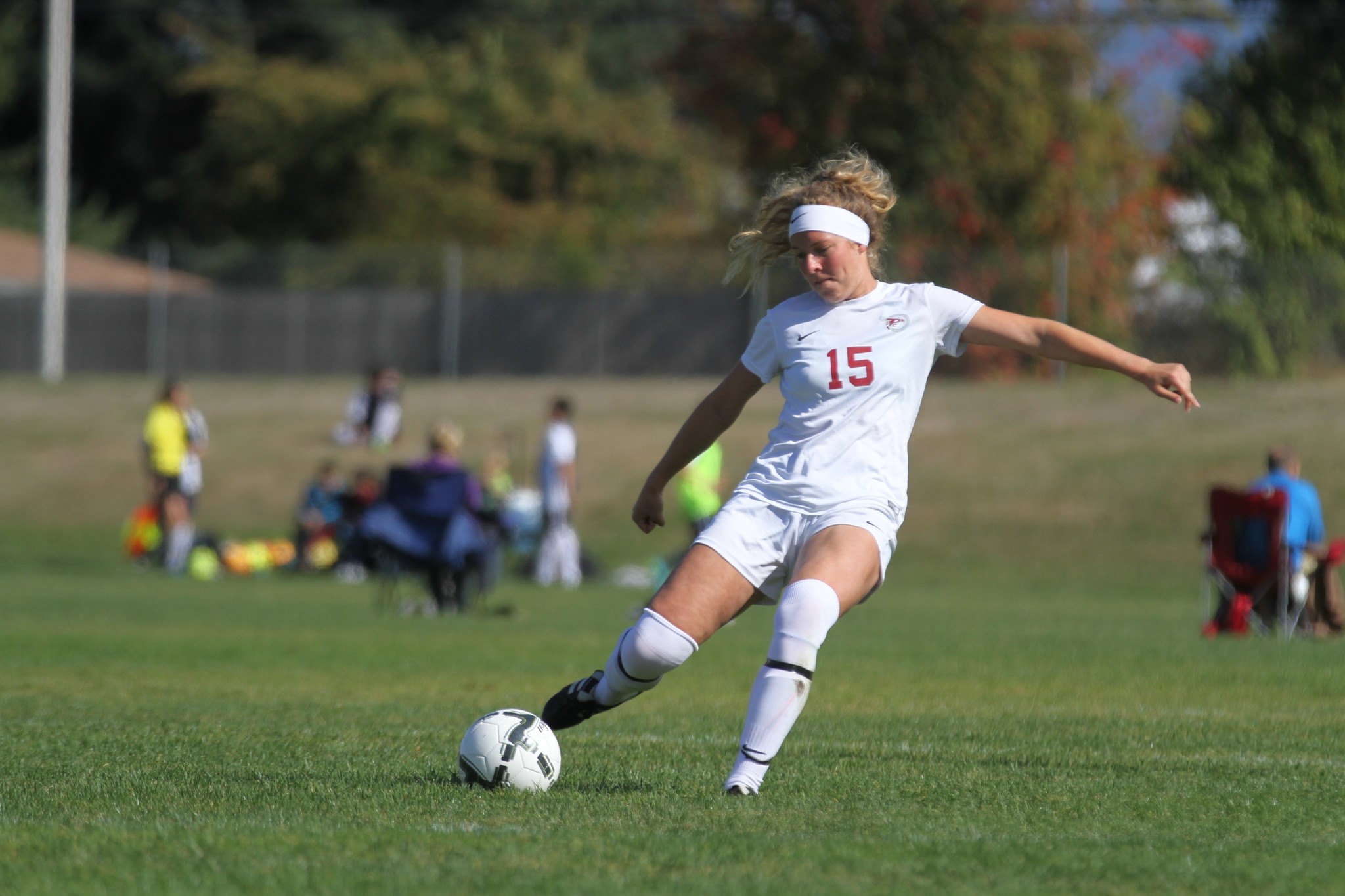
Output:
[144, 360, 726, 616]
[1252, 441, 1328, 637]
[543, 144, 1201, 797]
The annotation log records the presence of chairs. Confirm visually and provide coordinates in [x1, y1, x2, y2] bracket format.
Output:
[359, 465, 504, 619]
[1203, 485, 1345, 644]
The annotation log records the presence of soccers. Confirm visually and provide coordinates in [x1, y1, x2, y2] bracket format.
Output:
[460, 708, 561, 793]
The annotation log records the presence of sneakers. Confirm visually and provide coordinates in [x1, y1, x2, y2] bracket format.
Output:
[727, 784, 757, 797]
[541, 669, 642, 730]
